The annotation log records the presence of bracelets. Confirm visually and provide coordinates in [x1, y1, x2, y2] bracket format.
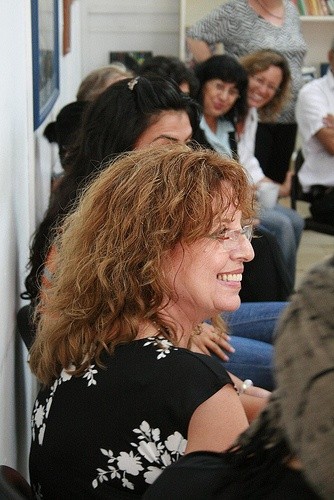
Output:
[240, 379, 252, 394]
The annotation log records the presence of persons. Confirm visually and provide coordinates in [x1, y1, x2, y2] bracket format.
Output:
[143, 258, 334, 500]
[29, 147, 254, 500]
[42, 0, 334, 302]
[24, 79, 288, 392]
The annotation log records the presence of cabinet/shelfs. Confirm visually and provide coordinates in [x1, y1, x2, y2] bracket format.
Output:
[180, 1, 333, 73]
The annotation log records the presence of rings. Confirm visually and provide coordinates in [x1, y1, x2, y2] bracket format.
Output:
[212, 334, 217, 342]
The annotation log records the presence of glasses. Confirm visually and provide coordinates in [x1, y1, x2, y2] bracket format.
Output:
[204, 225, 253, 242]
[250, 74, 282, 92]
[206, 81, 241, 97]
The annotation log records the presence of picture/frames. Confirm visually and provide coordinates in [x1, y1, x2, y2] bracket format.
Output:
[31, 0, 62, 133]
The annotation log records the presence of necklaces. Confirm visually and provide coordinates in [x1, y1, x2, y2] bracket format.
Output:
[257, 1, 282, 19]
